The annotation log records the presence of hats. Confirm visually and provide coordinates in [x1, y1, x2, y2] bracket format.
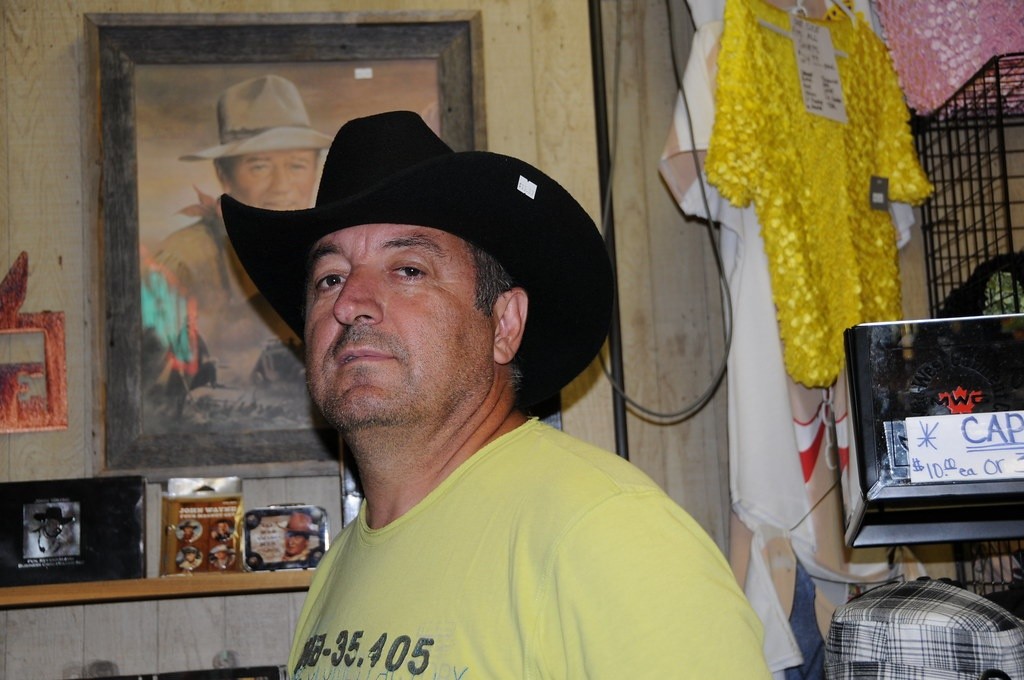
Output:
[220, 112, 616, 416]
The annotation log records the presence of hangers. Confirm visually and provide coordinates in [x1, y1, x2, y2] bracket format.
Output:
[757, 0, 854, 59]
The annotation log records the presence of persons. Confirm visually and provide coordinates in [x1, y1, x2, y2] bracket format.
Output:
[211, 544, 232, 569]
[276, 513, 320, 561]
[214, 520, 232, 542]
[220, 112, 772, 680]
[142, 75, 334, 385]
[33, 507, 75, 556]
[178, 546, 201, 570]
[179, 521, 198, 542]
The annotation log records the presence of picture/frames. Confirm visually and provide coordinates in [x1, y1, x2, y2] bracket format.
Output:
[82, 9, 491, 485]
